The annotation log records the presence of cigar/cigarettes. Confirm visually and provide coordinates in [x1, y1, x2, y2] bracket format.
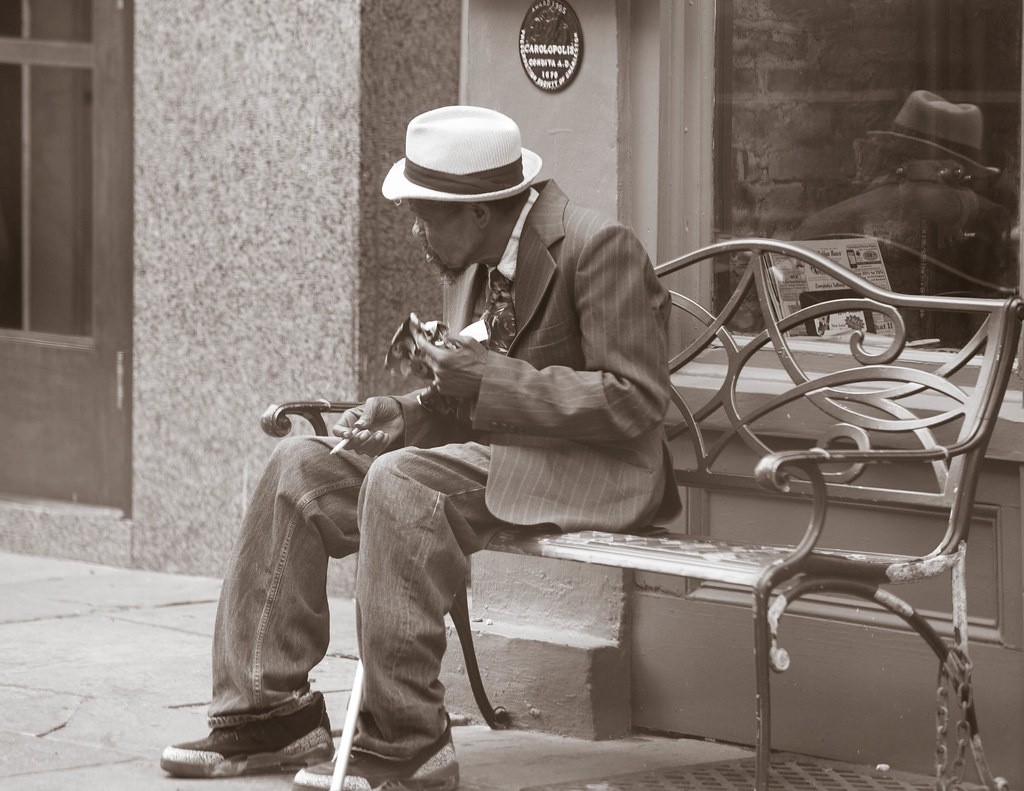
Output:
[330, 427, 360, 455]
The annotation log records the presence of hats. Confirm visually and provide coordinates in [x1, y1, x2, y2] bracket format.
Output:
[381, 105, 543, 203]
[866, 89, 1002, 175]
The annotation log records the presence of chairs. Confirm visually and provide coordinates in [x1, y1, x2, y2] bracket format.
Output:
[258, 238, 1023, 791]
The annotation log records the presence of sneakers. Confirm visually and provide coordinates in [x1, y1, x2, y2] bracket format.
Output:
[159, 691, 336, 780]
[294, 707, 459, 791]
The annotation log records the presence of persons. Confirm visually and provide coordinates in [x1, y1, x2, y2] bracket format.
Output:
[158, 105, 684, 791]
[786, 88, 1016, 349]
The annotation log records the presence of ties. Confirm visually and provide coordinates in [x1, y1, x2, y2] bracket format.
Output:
[382, 268, 518, 428]
